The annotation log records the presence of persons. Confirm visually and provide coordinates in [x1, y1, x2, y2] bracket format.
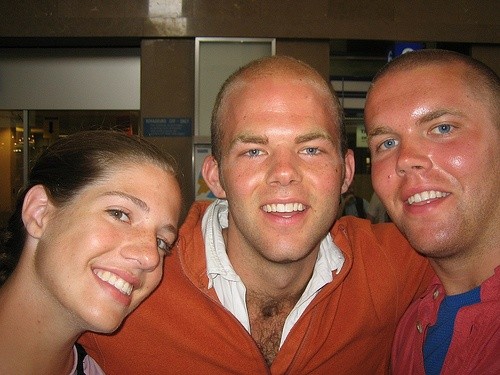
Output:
[365, 49, 500, 375]
[1, 130, 183, 375]
[76, 56, 440, 375]
